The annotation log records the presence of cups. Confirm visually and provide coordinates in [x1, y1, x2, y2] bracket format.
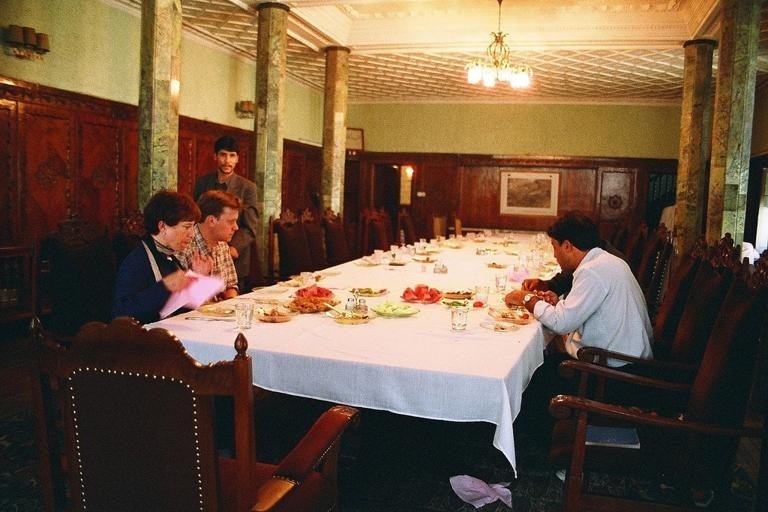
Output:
[519, 231, 549, 269]
[373, 249, 384, 259]
[435, 229, 477, 243]
[450, 307, 469, 332]
[476, 284, 491, 304]
[391, 245, 399, 252]
[235, 302, 254, 330]
[419, 238, 426, 243]
[300, 272, 316, 287]
[495, 273, 507, 287]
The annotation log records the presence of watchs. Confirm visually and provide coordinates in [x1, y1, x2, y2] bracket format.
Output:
[522, 293, 536, 307]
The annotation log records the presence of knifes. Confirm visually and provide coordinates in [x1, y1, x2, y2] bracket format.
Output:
[185, 316, 236, 323]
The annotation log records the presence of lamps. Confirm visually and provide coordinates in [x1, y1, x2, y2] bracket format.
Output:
[463, 1, 535, 91]
[237, 97, 255, 121]
[4, 20, 53, 65]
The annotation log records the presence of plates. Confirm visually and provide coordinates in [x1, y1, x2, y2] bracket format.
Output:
[442, 286, 476, 298]
[252, 286, 289, 295]
[483, 319, 524, 334]
[439, 297, 489, 310]
[402, 292, 443, 303]
[373, 303, 419, 316]
[326, 307, 376, 323]
[351, 284, 389, 299]
[489, 261, 508, 269]
[259, 313, 293, 323]
[201, 303, 234, 317]
[489, 307, 534, 324]
[282, 299, 342, 314]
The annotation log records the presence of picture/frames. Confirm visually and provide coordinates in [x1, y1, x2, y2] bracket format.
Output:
[498, 169, 562, 218]
[345, 127, 365, 151]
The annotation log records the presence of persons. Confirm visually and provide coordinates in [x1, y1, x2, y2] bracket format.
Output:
[170, 190, 246, 308]
[113, 187, 212, 326]
[191, 134, 260, 295]
[452, 212, 656, 487]
[520, 208, 628, 356]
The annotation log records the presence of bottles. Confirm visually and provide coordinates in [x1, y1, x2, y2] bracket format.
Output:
[346, 297, 355, 311]
[358, 300, 367, 311]
